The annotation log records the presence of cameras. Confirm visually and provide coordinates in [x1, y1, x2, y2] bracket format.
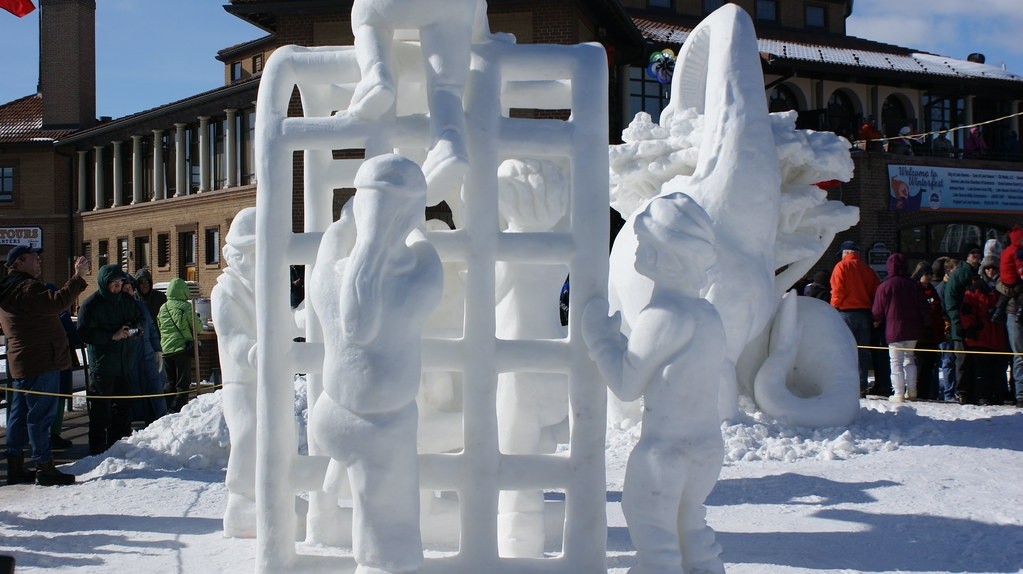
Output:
[125, 326, 138, 336]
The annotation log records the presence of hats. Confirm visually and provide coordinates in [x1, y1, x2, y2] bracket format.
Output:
[962, 242, 980, 262]
[978, 256, 1003, 275]
[7, 245, 44, 267]
[840, 241, 863, 253]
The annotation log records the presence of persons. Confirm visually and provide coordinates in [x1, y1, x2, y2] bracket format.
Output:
[581, 192, 728, 574]
[22, 264, 203, 450]
[210, 207, 306, 538]
[426, 155, 574, 558]
[0, 245, 90, 488]
[309, 152, 444, 574]
[775, 225, 1023, 408]
[855, 114, 1023, 162]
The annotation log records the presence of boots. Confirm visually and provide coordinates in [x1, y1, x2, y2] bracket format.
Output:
[6, 455, 36, 485]
[35, 457, 75, 486]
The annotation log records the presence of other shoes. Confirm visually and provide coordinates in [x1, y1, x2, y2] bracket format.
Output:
[1015, 315, 1023, 326]
[50, 435, 72, 449]
[888, 393, 904, 402]
[945, 396, 959, 403]
[990, 313, 1004, 324]
[904, 390, 918, 399]
[1016, 397, 1023, 408]
[959, 394, 966, 404]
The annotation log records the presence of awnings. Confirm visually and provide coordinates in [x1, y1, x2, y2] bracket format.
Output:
[629, 15, 1023, 80]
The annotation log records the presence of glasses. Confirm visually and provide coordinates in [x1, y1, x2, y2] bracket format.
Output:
[109, 281, 124, 285]
[138, 279, 150, 285]
[922, 271, 933, 276]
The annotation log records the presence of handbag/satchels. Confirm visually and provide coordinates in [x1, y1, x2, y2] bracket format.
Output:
[185, 340, 202, 358]
